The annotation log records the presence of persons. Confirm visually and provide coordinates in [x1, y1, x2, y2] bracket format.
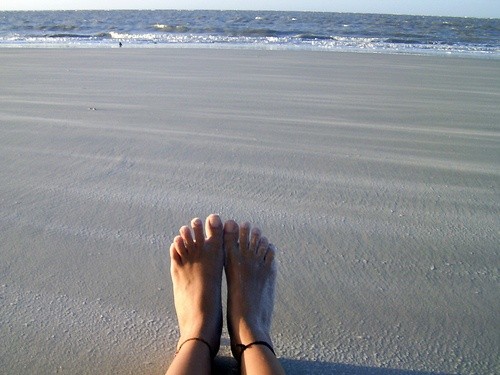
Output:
[169, 214, 287, 375]
